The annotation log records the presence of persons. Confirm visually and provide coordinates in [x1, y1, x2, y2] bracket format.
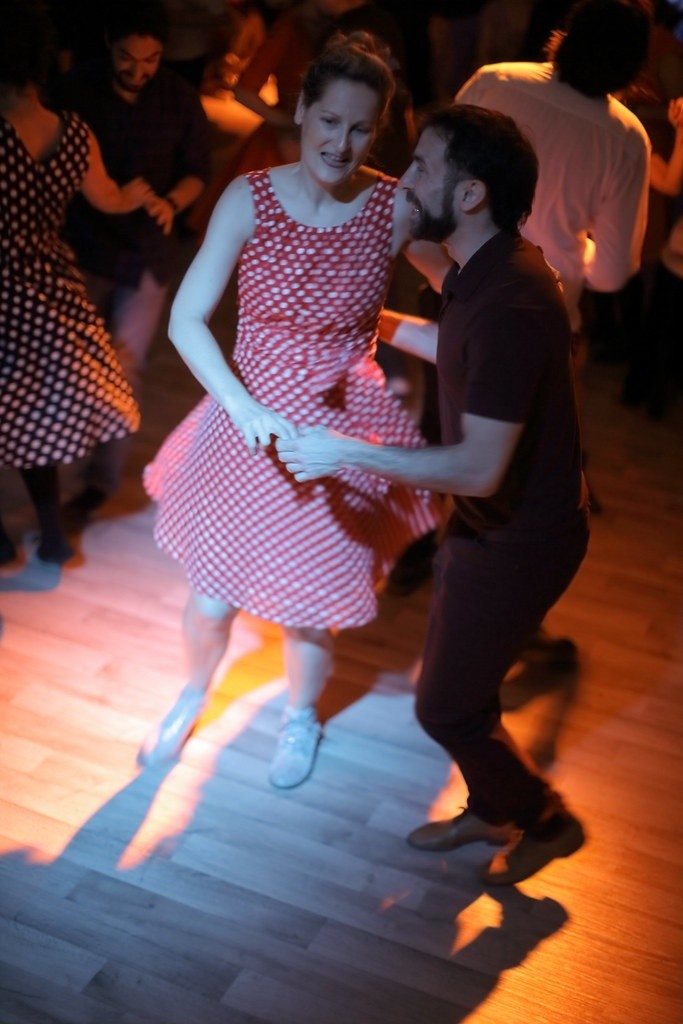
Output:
[0, 0, 154, 563]
[43, 1, 216, 506]
[231, 0, 683, 423]
[139, 30, 456, 787]
[276, 102, 588, 886]
[386, 1, 651, 598]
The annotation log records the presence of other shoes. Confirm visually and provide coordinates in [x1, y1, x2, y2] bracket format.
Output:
[1, 517, 72, 590]
[271, 712, 320, 789]
[137, 686, 204, 763]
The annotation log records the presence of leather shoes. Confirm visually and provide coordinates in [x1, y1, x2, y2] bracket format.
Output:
[406, 802, 521, 849]
[481, 818, 584, 887]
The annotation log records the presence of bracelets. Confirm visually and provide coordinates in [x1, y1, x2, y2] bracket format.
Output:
[165, 196, 179, 215]
